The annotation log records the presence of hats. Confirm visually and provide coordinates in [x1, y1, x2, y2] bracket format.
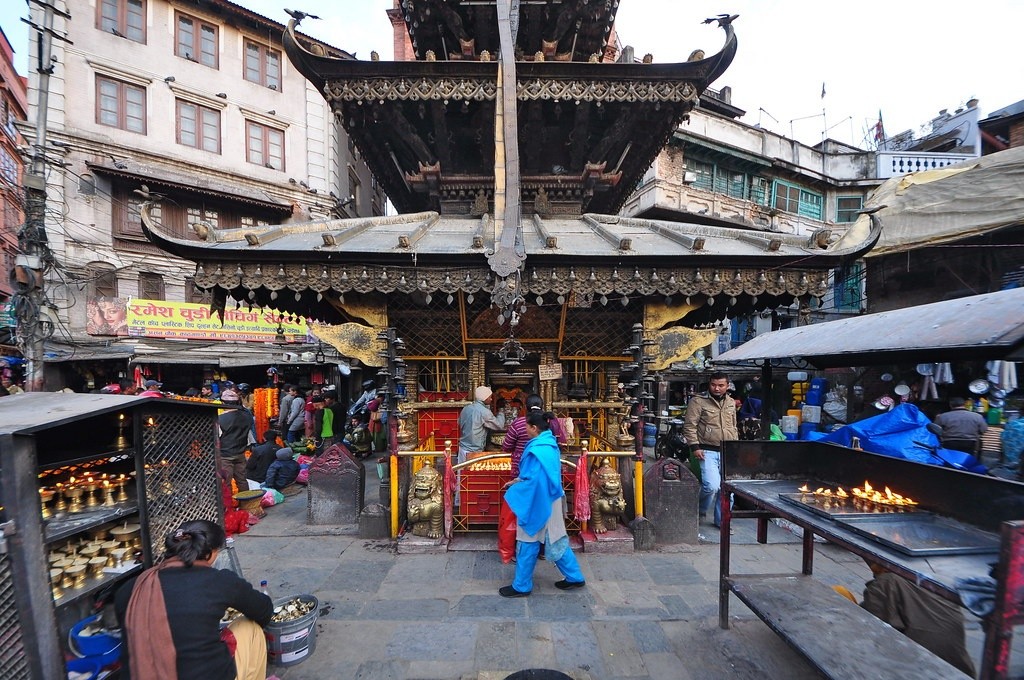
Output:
[221, 389, 239, 401]
[475, 385, 493, 401]
[276, 447, 293, 459]
[145, 380, 163, 388]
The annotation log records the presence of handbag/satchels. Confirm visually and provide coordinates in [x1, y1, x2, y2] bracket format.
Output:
[370, 398, 384, 423]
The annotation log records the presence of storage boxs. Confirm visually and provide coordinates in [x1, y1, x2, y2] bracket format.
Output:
[780, 377, 828, 434]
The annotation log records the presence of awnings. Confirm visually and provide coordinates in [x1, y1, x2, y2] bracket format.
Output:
[822, 144, 1024, 263]
[709, 284, 1024, 547]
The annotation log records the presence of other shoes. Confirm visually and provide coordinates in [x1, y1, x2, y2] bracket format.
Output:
[538, 554, 546, 560]
[700, 512, 706, 521]
[511, 556, 516, 565]
[718, 524, 734, 534]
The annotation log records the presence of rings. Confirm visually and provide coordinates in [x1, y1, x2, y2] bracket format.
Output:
[698, 457, 700, 459]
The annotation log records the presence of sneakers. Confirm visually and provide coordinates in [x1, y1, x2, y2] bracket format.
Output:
[498, 585, 531, 598]
[555, 578, 585, 590]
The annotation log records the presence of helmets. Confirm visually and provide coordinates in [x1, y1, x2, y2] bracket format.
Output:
[237, 383, 251, 393]
[362, 380, 375, 388]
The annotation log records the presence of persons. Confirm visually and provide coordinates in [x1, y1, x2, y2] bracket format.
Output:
[110, 516, 274, 680]
[683, 372, 740, 536]
[930, 396, 989, 457]
[0, 349, 395, 524]
[498, 409, 588, 598]
[499, 395, 558, 564]
[453, 385, 505, 511]
[999, 403, 1023, 470]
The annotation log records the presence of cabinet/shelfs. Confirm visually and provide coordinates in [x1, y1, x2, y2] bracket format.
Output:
[0, 390, 246, 679]
[717, 437, 1024, 680]
